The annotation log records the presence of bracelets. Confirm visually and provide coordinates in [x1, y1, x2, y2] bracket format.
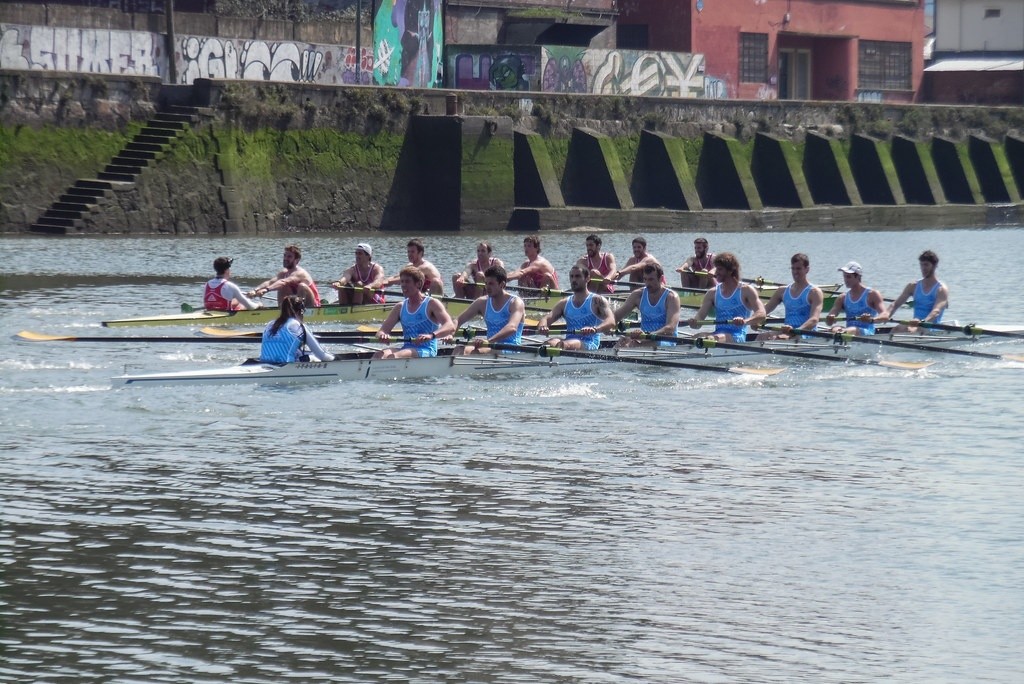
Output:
[870, 318, 873, 324]
[921, 319, 925, 322]
[336, 281, 341, 283]
[432, 332, 435, 339]
[617, 271, 621, 274]
[593, 327, 597, 333]
[266, 288, 269, 292]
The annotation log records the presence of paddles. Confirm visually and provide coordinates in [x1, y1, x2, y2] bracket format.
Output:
[888, 318, 1024, 338]
[200, 325, 580, 335]
[181, 292, 263, 313]
[590, 278, 771, 300]
[610, 328, 935, 370]
[766, 316, 860, 324]
[463, 281, 700, 310]
[336, 284, 551, 313]
[675, 269, 914, 308]
[16, 329, 418, 343]
[756, 324, 1001, 359]
[450, 337, 788, 376]
[616, 320, 735, 329]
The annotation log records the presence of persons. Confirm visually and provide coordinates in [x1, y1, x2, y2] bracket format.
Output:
[614, 262, 680, 347]
[577, 235, 617, 293]
[442, 265, 526, 355]
[886, 250, 949, 333]
[755, 253, 824, 340]
[616, 236, 666, 290]
[249, 246, 321, 307]
[826, 262, 889, 336]
[260, 295, 342, 362]
[689, 252, 767, 343]
[452, 243, 505, 300]
[371, 266, 456, 359]
[506, 236, 560, 298]
[383, 239, 444, 295]
[203, 256, 263, 311]
[537, 263, 616, 350]
[677, 238, 718, 289]
[332, 243, 386, 305]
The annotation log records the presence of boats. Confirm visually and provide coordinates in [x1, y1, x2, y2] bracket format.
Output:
[109, 324, 1020, 391]
[101, 289, 846, 329]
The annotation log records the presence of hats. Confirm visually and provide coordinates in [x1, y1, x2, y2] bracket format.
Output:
[356, 243, 372, 255]
[213, 257, 232, 271]
[838, 261, 862, 276]
